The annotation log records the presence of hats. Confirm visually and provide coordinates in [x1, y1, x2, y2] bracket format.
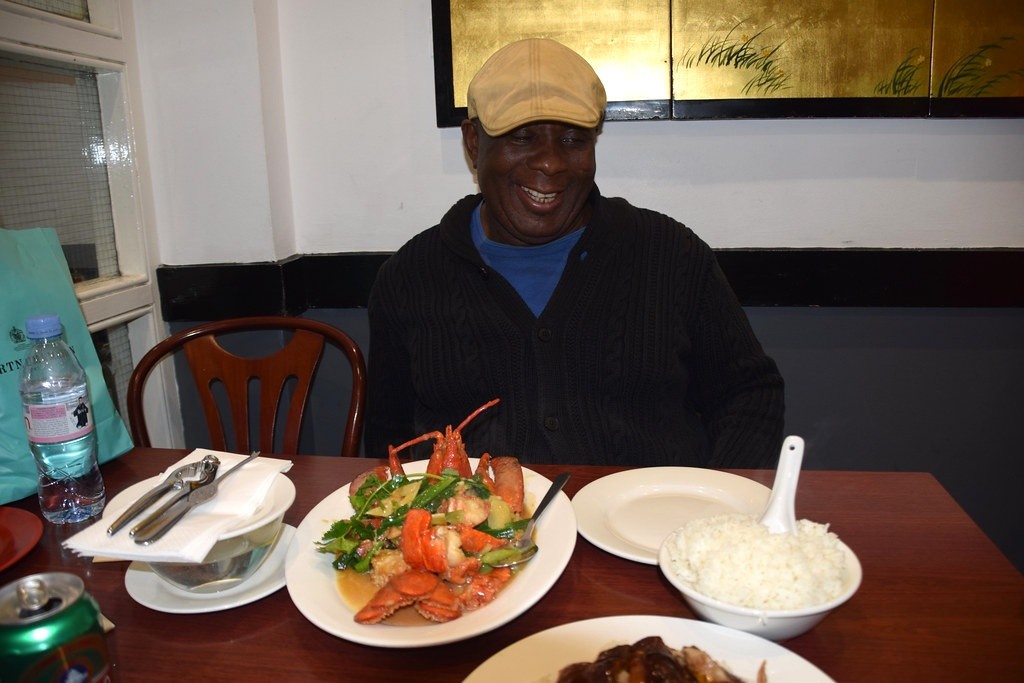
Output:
[467, 38, 606, 137]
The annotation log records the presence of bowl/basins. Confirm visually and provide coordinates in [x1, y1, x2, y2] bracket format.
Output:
[658, 516, 863, 640]
[136, 474, 297, 592]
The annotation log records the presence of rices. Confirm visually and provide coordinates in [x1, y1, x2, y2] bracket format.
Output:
[663, 511, 851, 614]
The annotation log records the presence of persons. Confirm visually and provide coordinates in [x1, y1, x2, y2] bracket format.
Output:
[360, 35, 790, 470]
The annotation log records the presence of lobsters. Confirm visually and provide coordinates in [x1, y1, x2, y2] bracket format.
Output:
[353, 397, 526, 627]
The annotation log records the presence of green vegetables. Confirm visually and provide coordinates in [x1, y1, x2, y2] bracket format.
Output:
[312, 466, 532, 573]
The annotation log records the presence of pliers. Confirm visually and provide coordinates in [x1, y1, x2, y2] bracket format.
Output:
[106, 454, 221, 539]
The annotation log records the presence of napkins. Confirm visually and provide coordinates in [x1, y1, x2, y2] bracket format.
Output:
[62, 449, 294, 563]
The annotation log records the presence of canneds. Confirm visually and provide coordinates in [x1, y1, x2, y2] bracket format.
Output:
[0, 572, 113, 683]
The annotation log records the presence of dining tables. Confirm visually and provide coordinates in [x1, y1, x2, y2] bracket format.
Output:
[0, 448, 1024, 683]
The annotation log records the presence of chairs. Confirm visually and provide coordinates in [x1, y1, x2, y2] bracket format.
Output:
[126, 316, 367, 458]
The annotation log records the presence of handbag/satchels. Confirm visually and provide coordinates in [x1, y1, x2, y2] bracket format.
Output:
[0, 228, 135, 506]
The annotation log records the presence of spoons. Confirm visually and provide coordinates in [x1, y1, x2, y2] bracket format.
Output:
[490, 473, 571, 568]
[758, 435, 805, 535]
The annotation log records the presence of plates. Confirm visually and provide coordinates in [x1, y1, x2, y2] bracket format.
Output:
[0, 508, 42, 571]
[124, 522, 298, 615]
[283, 459, 578, 649]
[461, 615, 836, 683]
[573, 467, 780, 568]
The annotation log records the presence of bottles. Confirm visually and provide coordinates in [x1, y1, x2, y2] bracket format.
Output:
[18, 315, 106, 525]
